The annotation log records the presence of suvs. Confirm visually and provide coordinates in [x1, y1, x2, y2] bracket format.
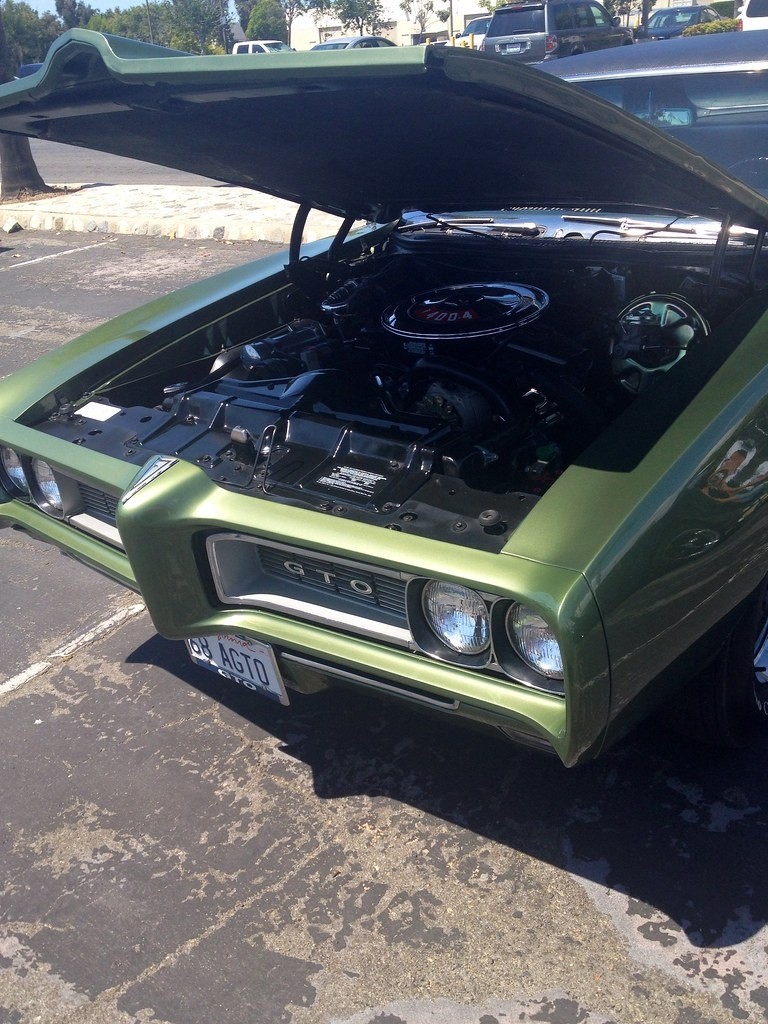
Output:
[480, 0, 634, 66]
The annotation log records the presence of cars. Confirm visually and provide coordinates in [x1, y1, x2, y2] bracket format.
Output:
[307, 35, 397, 51]
[455, 14, 493, 50]
[231, 39, 296, 54]
[633, 4, 722, 43]
[19, 61, 43, 77]
[736, 0, 768, 32]
[0, 27, 768, 774]
